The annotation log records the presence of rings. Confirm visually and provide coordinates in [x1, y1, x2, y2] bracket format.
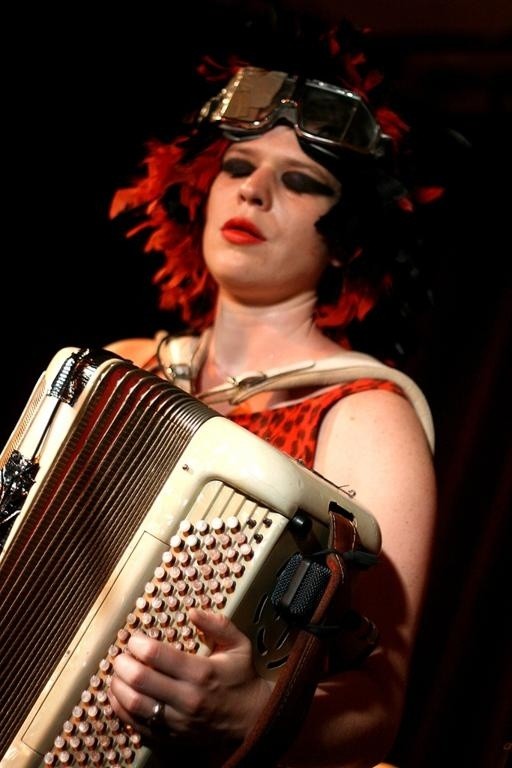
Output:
[152, 701, 163, 726]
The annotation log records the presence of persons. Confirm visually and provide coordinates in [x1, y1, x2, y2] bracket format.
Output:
[0, 65, 437, 768]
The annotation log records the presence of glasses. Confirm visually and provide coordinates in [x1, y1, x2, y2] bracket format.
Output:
[197, 64, 392, 163]
